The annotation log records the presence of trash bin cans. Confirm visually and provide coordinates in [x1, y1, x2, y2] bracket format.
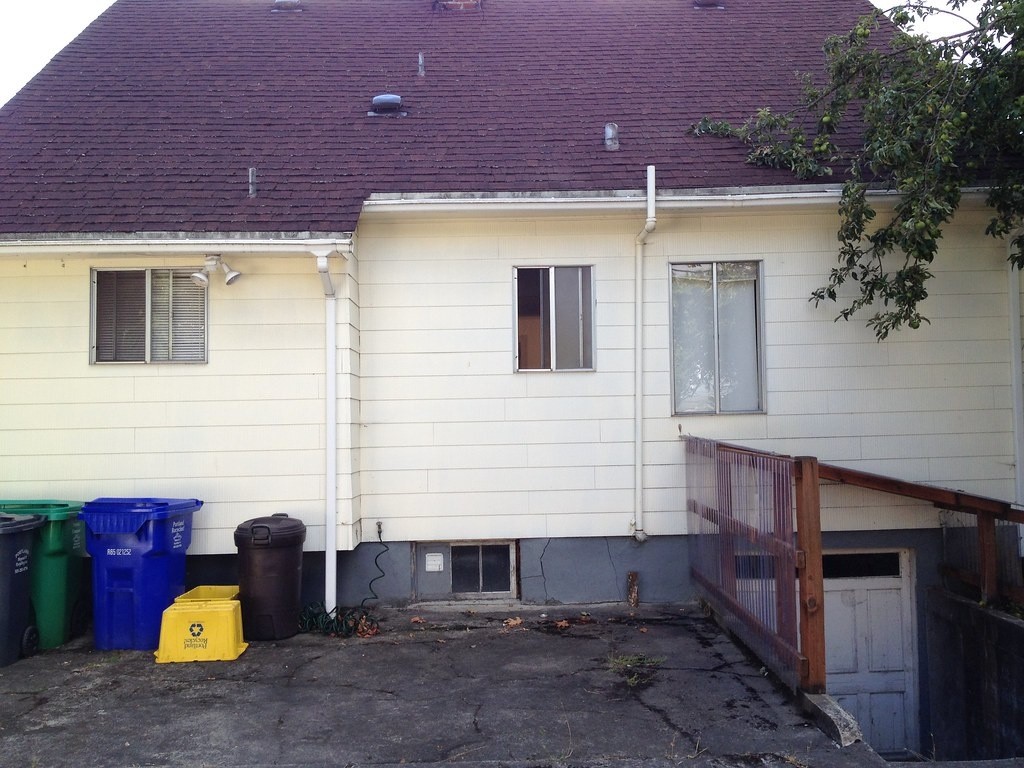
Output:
[234, 512, 307, 641]
[0, 496, 88, 668]
[77, 496, 203, 653]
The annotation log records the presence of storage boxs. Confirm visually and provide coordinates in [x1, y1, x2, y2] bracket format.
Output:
[156, 584, 249, 665]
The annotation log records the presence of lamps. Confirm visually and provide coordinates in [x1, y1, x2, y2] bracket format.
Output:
[191, 254, 242, 288]
